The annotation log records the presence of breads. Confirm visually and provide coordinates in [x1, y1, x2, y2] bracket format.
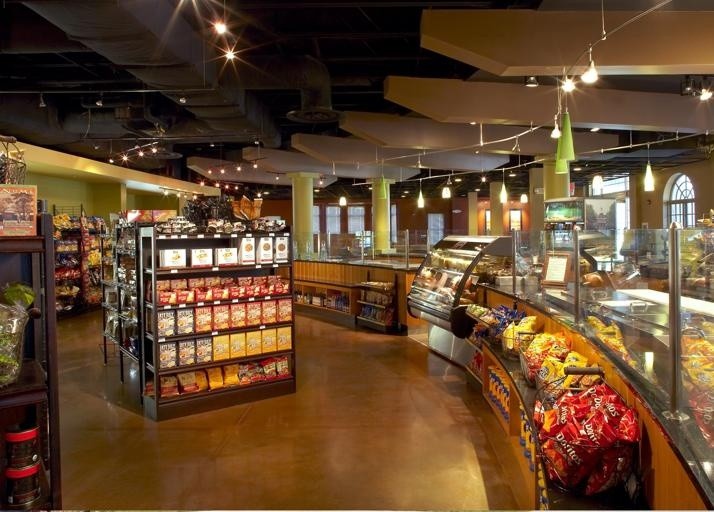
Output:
[429, 256, 489, 273]
[647, 264, 690, 292]
[582, 273, 603, 287]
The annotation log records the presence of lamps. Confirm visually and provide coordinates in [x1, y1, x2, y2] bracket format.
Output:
[526, 75, 539, 88]
[555, 93, 575, 174]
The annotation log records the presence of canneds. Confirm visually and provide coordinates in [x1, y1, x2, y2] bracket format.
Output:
[4, 420, 43, 507]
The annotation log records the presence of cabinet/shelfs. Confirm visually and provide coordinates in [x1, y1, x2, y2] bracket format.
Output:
[0, 201, 60, 511]
[98, 213, 297, 420]
[292, 230, 421, 336]
[466, 220, 714, 509]
[407, 234, 514, 366]
[50, 208, 108, 314]
